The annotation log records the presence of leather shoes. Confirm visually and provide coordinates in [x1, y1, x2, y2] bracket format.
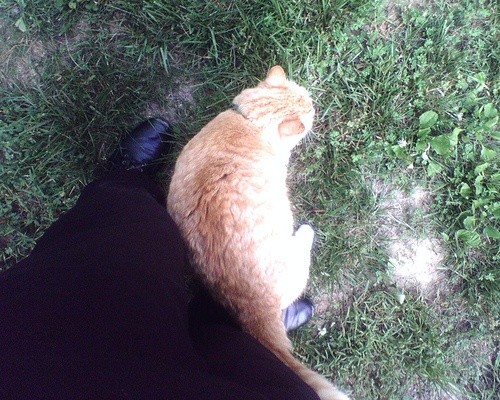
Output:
[281, 296, 316, 332]
[103, 118, 173, 178]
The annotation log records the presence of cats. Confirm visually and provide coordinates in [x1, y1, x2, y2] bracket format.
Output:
[165, 64, 351, 400]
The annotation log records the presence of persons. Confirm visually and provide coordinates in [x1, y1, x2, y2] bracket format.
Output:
[0, 117, 321, 400]
